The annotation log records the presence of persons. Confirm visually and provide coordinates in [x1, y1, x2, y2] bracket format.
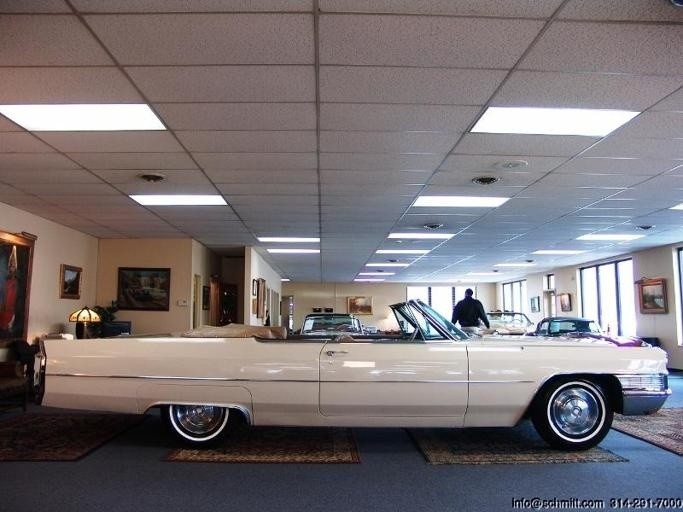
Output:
[447, 287, 490, 339]
[0, 257, 21, 336]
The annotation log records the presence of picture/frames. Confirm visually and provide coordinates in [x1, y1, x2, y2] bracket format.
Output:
[347, 296, 373, 315]
[253, 279, 257, 296]
[0, 230, 35, 350]
[60, 263, 82, 299]
[635, 279, 668, 314]
[530, 296, 539, 313]
[202, 285, 211, 311]
[117, 267, 170, 311]
[560, 293, 572, 311]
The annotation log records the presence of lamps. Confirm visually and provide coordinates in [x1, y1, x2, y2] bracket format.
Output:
[68, 305, 101, 339]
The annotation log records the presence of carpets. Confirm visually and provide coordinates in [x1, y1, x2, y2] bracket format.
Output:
[409, 428, 629, 467]
[2, 410, 109, 462]
[162, 425, 361, 475]
[610, 407, 683, 455]
